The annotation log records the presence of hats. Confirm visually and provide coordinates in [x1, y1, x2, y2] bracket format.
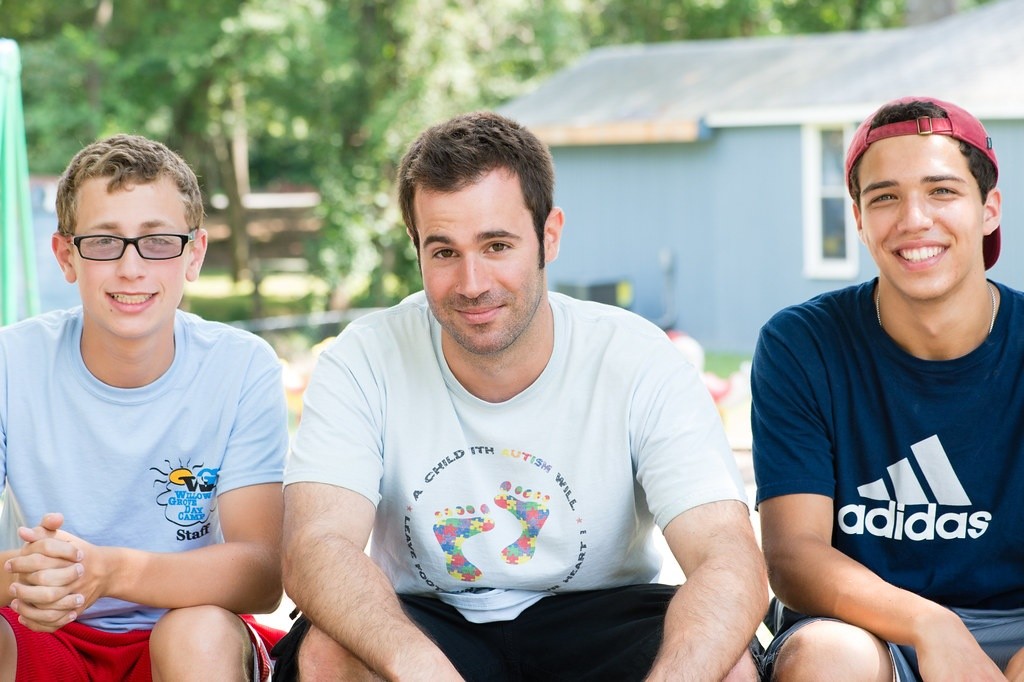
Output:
[846, 97, 1004, 272]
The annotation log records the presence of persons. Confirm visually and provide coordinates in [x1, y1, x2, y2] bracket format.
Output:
[0, 133, 288, 682]
[281, 113, 773, 682]
[746, 95, 1024, 682]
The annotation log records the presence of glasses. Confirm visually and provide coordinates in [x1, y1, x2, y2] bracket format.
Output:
[63, 229, 195, 261]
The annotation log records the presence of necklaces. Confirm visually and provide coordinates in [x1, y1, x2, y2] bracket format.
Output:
[876, 282, 996, 339]
